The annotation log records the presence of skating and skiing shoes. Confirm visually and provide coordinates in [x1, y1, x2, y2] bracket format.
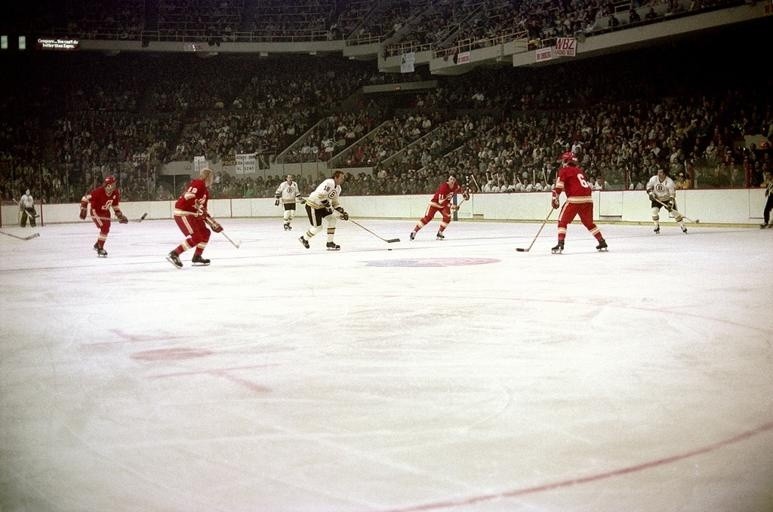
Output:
[552, 239, 564, 254]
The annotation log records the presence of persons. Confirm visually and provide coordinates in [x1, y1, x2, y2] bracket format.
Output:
[0, 0, 773, 205]
[298, 171, 348, 251]
[274, 174, 306, 231]
[409, 175, 469, 242]
[646, 168, 687, 235]
[550, 152, 607, 254]
[19, 186, 35, 227]
[164, 168, 222, 269]
[79, 176, 128, 258]
[758, 172, 773, 229]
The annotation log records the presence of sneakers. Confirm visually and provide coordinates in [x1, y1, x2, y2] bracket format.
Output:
[436, 230, 445, 240]
[93, 241, 108, 258]
[326, 241, 340, 251]
[595, 239, 608, 252]
[653, 224, 660, 234]
[760, 221, 769, 229]
[192, 253, 211, 266]
[166, 250, 183, 269]
[298, 235, 310, 249]
[680, 224, 688, 234]
[409, 231, 416, 241]
[284, 222, 292, 231]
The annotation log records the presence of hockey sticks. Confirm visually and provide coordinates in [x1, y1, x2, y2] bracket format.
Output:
[442, 197, 466, 217]
[515, 207, 554, 252]
[13, 198, 40, 219]
[0, 231, 40, 240]
[348, 218, 400, 243]
[655, 198, 699, 225]
[205, 217, 241, 249]
[86, 212, 148, 222]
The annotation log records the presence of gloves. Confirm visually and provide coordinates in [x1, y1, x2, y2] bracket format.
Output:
[184, 193, 208, 220]
[333, 206, 349, 221]
[204, 213, 224, 233]
[667, 196, 675, 213]
[646, 187, 656, 201]
[320, 198, 333, 215]
[446, 202, 460, 211]
[79, 199, 90, 221]
[551, 187, 564, 210]
[113, 206, 128, 225]
[462, 190, 470, 201]
[296, 193, 307, 204]
[765, 181, 773, 198]
[274, 193, 281, 207]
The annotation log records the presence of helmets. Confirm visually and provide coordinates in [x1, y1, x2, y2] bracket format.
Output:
[102, 175, 117, 188]
[556, 151, 578, 165]
